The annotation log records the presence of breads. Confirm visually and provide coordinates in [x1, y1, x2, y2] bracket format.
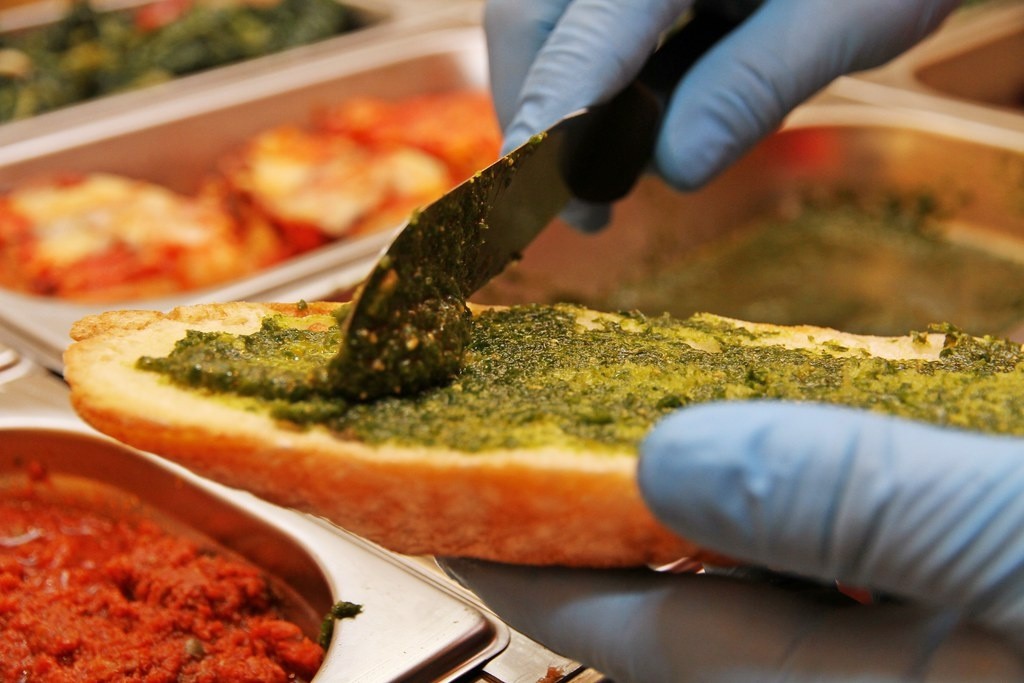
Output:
[65, 296, 1024, 595]
[1, 80, 506, 300]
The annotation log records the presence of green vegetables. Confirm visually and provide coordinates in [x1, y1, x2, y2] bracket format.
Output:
[0, 0, 348, 127]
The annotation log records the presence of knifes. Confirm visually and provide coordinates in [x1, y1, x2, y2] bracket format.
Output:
[329, 0, 767, 350]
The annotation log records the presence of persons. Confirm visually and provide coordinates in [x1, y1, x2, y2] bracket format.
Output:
[483, 0, 1024, 655]
